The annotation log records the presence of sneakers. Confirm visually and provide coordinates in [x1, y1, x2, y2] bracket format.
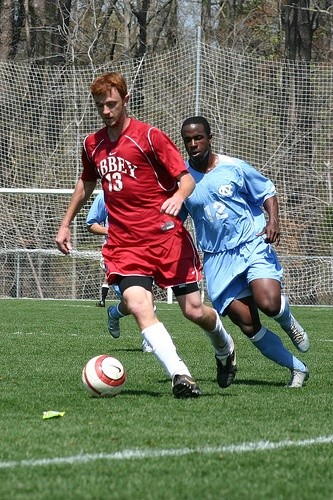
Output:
[285, 361, 310, 388]
[280, 313, 310, 353]
[213, 334, 238, 389]
[171, 373, 203, 397]
[106, 305, 121, 339]
[95, 301, 106, 307]
[140, 338, 153, 353]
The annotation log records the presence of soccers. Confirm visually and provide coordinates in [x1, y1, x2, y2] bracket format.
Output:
[81, 354, 126, 398]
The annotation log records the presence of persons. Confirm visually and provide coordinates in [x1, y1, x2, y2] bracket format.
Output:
[178, 116, 311, 388]
[55, 72, 237, 399]
